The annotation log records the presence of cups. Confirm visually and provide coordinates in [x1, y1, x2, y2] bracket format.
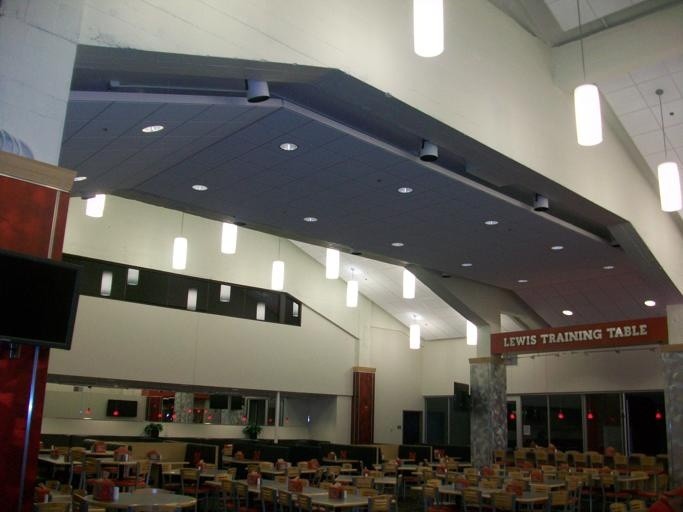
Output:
[111, 486, 119, 501]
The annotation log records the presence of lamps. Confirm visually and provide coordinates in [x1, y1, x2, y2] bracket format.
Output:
[650, 87, 683, 214]
[566, 1, 606, 148]
[416, 138, 439, 162]
[410, 0, 446, 59]
[533, 188, 550, 211]
[243, 77, 274, 106]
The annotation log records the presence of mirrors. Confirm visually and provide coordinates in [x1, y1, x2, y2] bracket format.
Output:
[43, 373, 285, 428]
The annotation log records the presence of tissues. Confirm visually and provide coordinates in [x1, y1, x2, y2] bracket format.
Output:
[35, 440, 617, 503]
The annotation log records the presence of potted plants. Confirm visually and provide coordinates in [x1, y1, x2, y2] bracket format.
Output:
[241, 423, 261, 439]
[143, 423, 163, 438]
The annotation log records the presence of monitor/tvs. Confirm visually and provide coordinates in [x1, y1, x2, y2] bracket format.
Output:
[453, 381, 469, 411]
[0, 248, 83, 351]
